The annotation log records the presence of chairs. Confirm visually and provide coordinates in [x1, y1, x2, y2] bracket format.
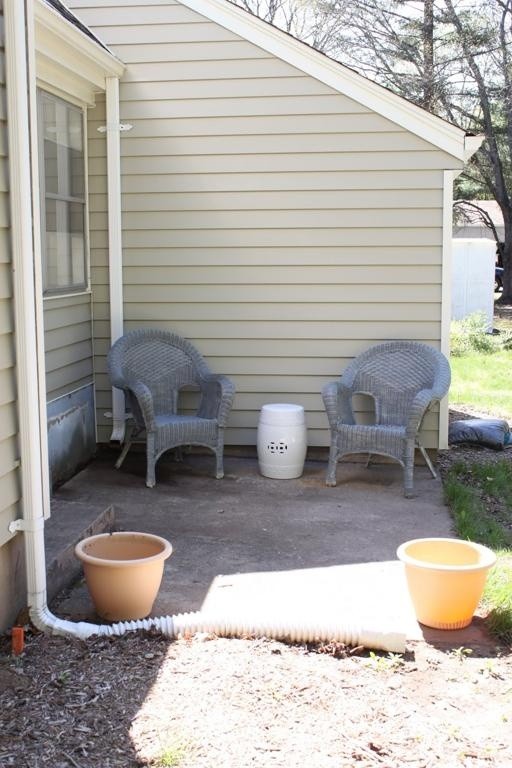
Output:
[104, 324, 234, 487]
[323, 341, 449, 488]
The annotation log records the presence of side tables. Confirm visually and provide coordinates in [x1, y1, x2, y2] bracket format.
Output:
[253, 401, 310, 479]
